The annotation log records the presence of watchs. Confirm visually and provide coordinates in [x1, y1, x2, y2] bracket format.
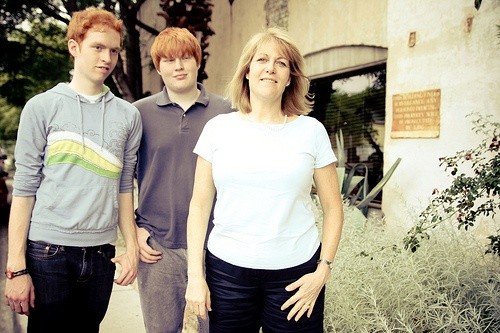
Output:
[5, 269, 30, 279]
[317, 259, 333, 270]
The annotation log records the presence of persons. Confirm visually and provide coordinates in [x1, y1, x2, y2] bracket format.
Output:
[185, 27, 344, 333]
[5, 6, 143, 333]
[132, 27, 239, 333]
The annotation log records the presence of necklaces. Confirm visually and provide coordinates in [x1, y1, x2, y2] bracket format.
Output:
[247, 110, 288, 131]
